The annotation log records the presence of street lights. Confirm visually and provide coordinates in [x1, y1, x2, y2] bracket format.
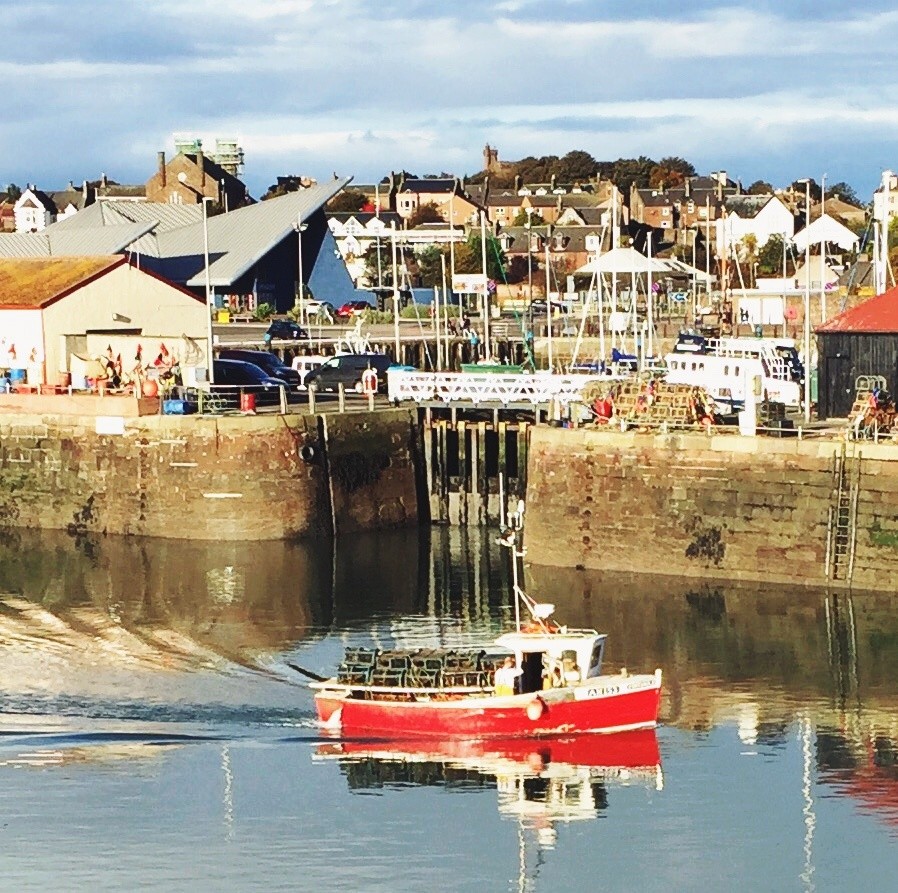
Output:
[798, 173, 830, 423]
[202, 197, 215, 382]
[292, 213, 309, 325]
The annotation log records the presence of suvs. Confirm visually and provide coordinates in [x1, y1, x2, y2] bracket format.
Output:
[220, 349, 301, 390]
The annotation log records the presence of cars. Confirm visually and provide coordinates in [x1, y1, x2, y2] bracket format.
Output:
[338, 300, 371, 318]
[291, 298, 335, 316]
[267, 319, 308, 340]
[213, 360, 290, 405]
[775, 346, 802, 379]
[528, 298, 567, 314]
[673, 335, 705, 355]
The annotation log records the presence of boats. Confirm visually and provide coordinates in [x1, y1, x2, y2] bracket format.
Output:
[309, 472, 664, 771]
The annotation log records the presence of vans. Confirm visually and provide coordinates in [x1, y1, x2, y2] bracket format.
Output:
[291, 355, 392, 394]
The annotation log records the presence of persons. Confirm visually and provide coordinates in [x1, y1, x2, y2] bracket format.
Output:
[552, 667, 567, 688]
[494, 657, 523, 696]
[463, 315, 471, 338]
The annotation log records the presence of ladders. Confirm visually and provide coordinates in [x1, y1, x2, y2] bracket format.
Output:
[825, 450, 862, 582]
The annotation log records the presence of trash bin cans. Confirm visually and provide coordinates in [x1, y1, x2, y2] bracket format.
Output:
[700, 327, 720, 351]
[460, 364, 521, 375]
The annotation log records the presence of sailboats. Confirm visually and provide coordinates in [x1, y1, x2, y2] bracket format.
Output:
[385, 187, 787, 372]
